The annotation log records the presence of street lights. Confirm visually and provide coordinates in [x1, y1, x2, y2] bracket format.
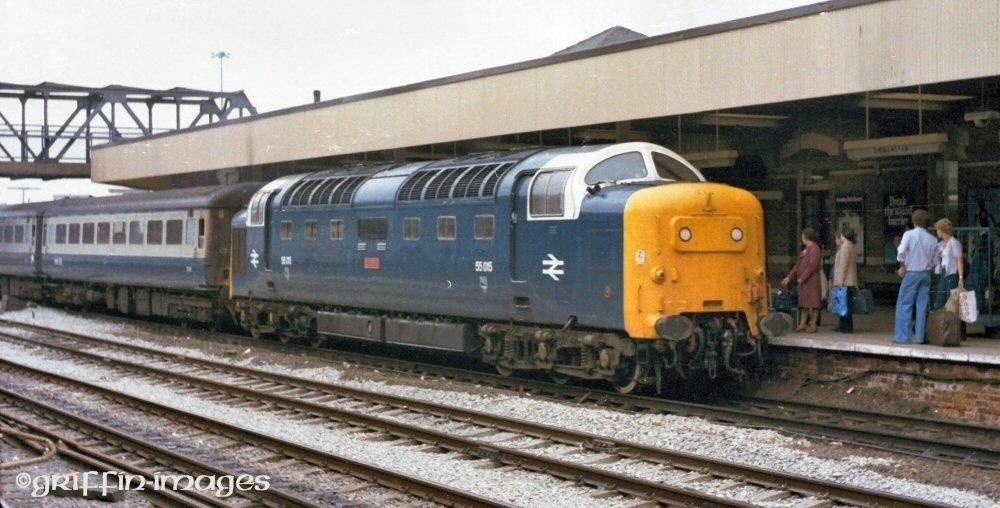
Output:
[211, 50, 231, 109]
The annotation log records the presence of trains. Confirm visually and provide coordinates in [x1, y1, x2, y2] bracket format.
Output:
[0, 140, 794, 399]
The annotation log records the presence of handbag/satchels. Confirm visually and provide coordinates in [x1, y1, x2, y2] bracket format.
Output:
[773, 290, 796, 310]
[853, 289, 875, 314]
[832, 287, 848, 316]
[925, 288, 977, 346]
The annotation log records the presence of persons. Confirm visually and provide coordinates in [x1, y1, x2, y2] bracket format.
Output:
[891, 209, 969, 344]
[780, 225, 858, 334]
[973, 198, 997, 229]
